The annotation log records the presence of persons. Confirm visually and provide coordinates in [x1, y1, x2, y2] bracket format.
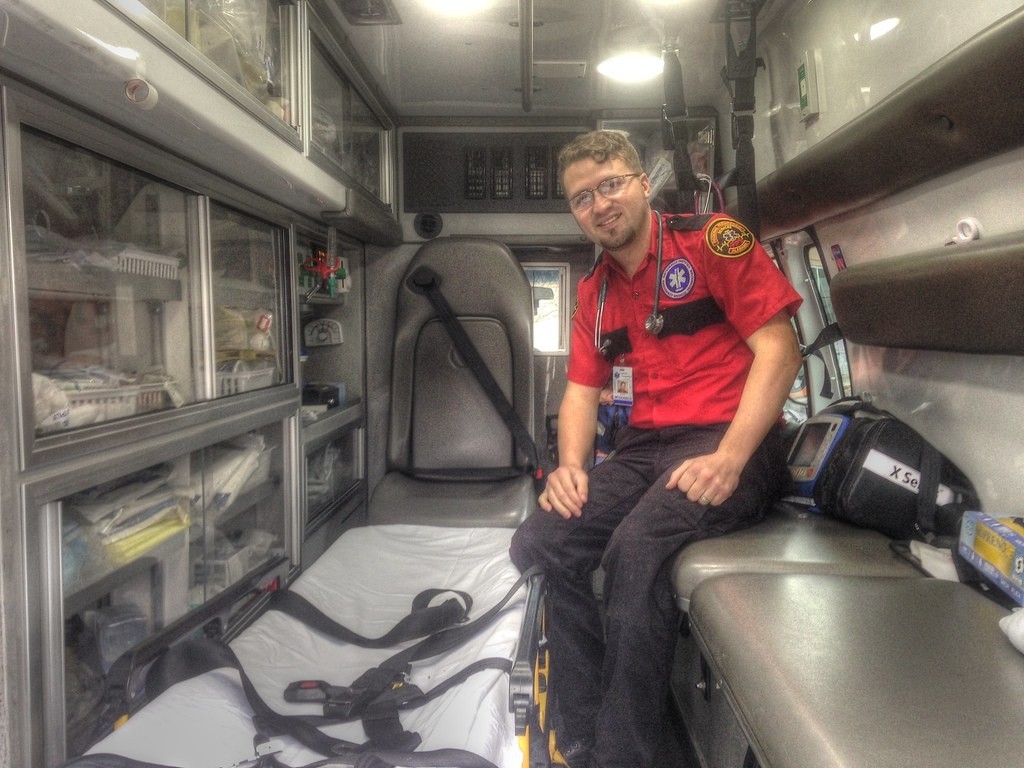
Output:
[618, 382, 627, 393]
[509, 130, 802, 767]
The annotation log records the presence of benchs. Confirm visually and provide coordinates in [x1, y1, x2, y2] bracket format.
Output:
[664, 507, 1024, 768]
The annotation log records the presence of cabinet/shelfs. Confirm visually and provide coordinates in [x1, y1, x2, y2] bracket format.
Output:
[0, 1, 404, 768]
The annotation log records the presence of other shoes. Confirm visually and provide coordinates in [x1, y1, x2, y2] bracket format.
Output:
[560, 734, 596, 767]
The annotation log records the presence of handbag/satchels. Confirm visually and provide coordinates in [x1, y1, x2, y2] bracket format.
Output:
[780, 390, 985, 552]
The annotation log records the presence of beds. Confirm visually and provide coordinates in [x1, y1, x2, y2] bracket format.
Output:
[69, 522, 548, 768]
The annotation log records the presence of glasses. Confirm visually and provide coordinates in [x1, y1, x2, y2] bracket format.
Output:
[562, 174, 640, 213]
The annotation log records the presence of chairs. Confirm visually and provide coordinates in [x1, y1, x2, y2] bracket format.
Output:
[368, 236, 537, 527]
